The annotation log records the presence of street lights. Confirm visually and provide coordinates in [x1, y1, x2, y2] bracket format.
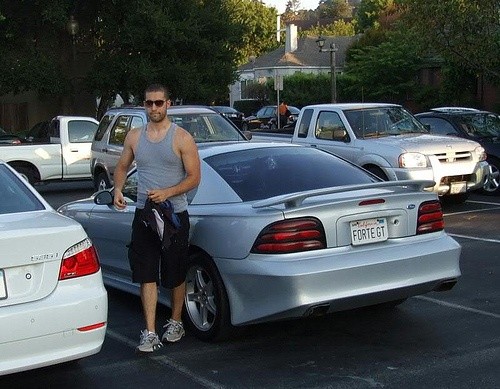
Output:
[315, 34, 338, 104]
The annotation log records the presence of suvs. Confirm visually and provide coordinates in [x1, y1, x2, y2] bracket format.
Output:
[88, 104, 254, 191]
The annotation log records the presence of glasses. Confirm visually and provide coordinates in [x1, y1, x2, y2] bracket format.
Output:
[144, 98, 171, 107]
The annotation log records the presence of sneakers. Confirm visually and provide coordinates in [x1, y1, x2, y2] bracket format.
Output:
[161, 319, 186, 345]
[135, 328, 165, 353]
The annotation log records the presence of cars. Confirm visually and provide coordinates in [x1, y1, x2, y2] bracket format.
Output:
[242, 115, 263, 130]
[0, 157, 110, 376]
[207, 106, 247, 133]
[255, 105, 302, 134]
[381, 106, 500, 199]
[56, 140, 456, 345]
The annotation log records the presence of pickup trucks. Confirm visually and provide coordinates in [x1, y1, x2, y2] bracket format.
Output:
[0, 114, 104, 191]
[251, 102, 491, 209]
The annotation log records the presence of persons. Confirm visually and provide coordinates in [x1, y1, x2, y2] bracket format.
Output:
[278, 99, 288, 127]
[115, 84, 200, 352]
